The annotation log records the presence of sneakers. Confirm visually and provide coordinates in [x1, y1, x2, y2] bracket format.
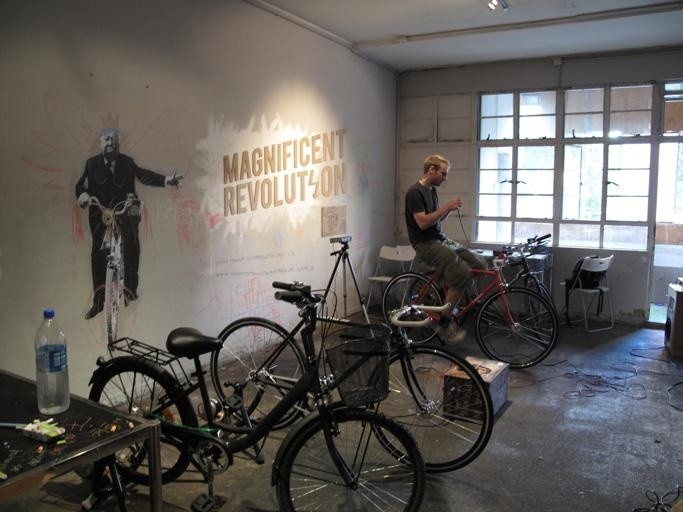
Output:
[445, 329, 465, 345]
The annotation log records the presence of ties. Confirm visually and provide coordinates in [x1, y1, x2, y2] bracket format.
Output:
[106, 161, 112, 170]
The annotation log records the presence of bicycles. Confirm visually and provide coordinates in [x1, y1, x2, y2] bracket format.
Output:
[382, 251, 559, 368]
[210, 293, 492, 472]
[88, 281, 426, 512]
[406, 234, 558, 348]
[86, 193, 143, 348]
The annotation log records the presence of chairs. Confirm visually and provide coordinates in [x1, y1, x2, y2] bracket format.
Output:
[366, 246, 415, 313]
[561, 255, 615, 332]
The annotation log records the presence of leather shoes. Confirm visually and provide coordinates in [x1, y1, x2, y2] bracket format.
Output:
[124, 294, 137, 306]
[85, 303, 103, 318]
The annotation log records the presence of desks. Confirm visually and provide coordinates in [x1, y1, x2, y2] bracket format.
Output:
[469, 249, 553, 325]
[0, 366, 163, 512]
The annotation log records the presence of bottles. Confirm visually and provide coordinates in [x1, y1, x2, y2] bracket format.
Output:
[35, 308, 70, 414]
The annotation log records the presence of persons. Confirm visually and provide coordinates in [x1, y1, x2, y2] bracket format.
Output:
[405, 155, 486, 341]
[75, 129, 185, 321]
